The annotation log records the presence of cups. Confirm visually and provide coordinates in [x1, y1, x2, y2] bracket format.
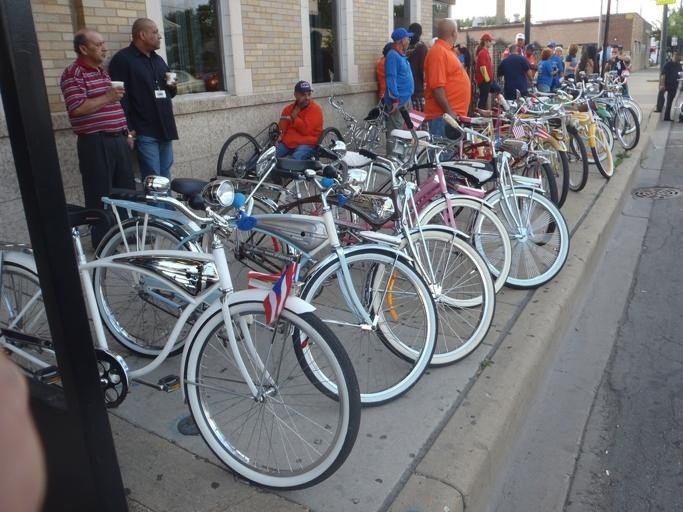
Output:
[111, 81, 124, 97]
[166, 72, 176, 85]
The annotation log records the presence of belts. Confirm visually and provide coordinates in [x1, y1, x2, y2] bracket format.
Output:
[98, 129, 130, 138]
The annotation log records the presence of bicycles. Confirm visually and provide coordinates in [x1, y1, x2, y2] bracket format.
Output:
[494, 70, 642, 209]
[274, 114, 512, 307]
[361, 129, 571, 290]
[90, 138, 440, 409]
[0, 144, 363, 492]
[329, 92, 389, 150]
[201, 150, 497, 369]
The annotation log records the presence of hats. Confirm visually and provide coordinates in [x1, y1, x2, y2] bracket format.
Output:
[527, 44, 538, 50]
[480, 32, 495, 41]
[391, 27, 415, 41]
[294, 80, 314, 93]
[516, 33, 525, 39]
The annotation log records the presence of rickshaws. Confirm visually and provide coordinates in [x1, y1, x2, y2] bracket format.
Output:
[217, 122, 346, 178]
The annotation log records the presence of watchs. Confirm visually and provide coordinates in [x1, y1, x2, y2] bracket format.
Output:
[126, 133, 133, 138]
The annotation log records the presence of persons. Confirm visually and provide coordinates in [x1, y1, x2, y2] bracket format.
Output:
[273, 80, 324, 185]
[61, 28, 156, 256]
[424, 18, 471, 201]
[565, 44, 580, 76]
[456, 44, 471, 73]
[377, 42, 391, 105]
[536, 48, 558, 92]
[384, 27, 414, 156]
[525, 44, 537, 80]
[408, 24, 430, 131]
[312, 31, 335, 82]
[109, 18, 178, 189]
[654, 48, 682, 121]
[548, 43, 564, 90]
[607, 45, 628, 97]
[475, 33, 494, 109]
[498, 45, 532, 100]
[515, 33, 524, 45]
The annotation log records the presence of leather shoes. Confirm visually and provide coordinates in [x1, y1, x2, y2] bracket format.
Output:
[121, 236, 155, 244]
